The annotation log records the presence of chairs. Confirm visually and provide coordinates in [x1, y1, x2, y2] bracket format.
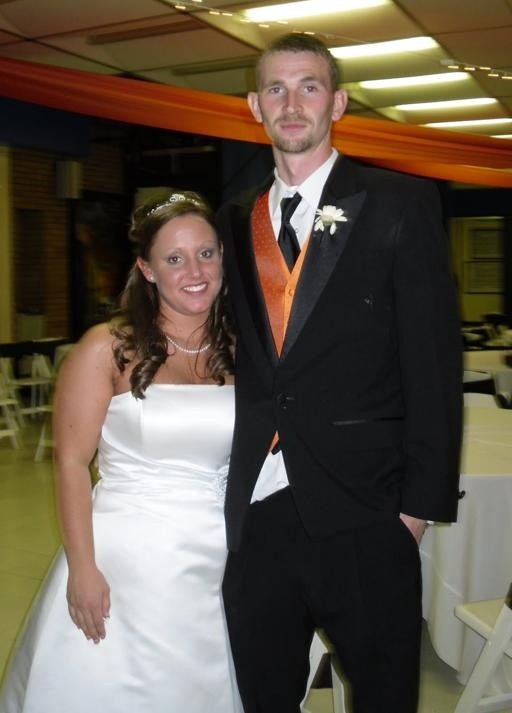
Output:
[455, 587, 511, 713]
[1, 342, 88, 463]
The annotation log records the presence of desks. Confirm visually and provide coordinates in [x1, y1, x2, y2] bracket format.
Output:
[416, 389, 512, 665]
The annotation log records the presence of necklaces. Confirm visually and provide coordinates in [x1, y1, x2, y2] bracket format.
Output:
[157, 330, 212, 354]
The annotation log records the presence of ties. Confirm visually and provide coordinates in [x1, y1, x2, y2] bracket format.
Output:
[277, 194, 303, 272]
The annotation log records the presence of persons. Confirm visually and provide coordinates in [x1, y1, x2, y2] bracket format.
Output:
[209, 34, 462, 713]
[2, 188, 248, 713]
